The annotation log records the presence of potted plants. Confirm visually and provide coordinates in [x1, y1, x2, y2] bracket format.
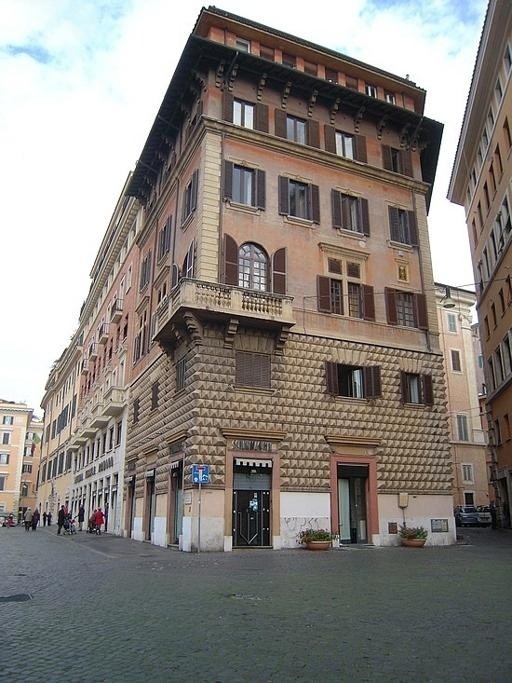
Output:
[398, 524, 429, 547]
[296, 527, 332, 550]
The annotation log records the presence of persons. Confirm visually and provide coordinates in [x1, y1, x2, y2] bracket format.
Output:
[48, 512, 52, 525]
[75, 502, 85, 532]
[104, 502, 109, 530]
[94, 507, 106, 534]
[56, 505, 65, 537]
[33, 509, 41, 532]
[43, 512, 48, 526]
[23, 507, 31, 530]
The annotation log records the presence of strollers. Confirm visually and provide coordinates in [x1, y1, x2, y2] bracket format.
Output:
[63, 515, 77, 535]
[86, 519, 95, 534]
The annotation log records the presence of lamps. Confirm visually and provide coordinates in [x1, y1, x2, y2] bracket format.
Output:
[442, 285, 456, 308]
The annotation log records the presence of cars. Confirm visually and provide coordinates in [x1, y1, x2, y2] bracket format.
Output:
[454, 505, 493, 528]
